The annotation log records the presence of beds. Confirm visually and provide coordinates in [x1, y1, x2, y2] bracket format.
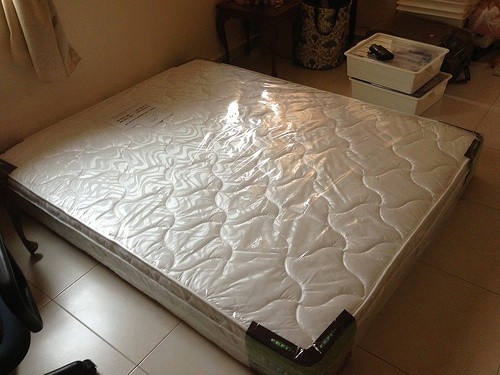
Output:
[0, 57, 483, 375]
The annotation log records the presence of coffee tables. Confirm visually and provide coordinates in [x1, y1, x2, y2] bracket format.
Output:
[215, 0, 302, 78]
[0, 160, 40, 254]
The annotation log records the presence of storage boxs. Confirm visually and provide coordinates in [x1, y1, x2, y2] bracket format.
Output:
[344, 32, 452, 116]
